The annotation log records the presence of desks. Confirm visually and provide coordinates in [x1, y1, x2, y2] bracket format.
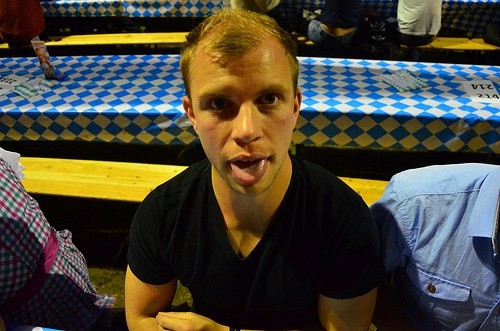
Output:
[0, 54, 500, 154]
[41, 1, 500, 35]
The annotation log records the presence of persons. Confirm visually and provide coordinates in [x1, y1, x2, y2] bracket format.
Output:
[386, 0, 444, 45]
[0, 0, 56, 80]
[305, 0, 371, 53]
[229, 0, 302, 30]
[124, 8, 382, 331]
[0, 158, 126, 331]
[370, 163, 500, 331]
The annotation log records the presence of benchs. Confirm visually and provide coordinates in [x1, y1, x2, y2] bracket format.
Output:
[18, 156, 389, 209]
[0, 33, 500, 51]
